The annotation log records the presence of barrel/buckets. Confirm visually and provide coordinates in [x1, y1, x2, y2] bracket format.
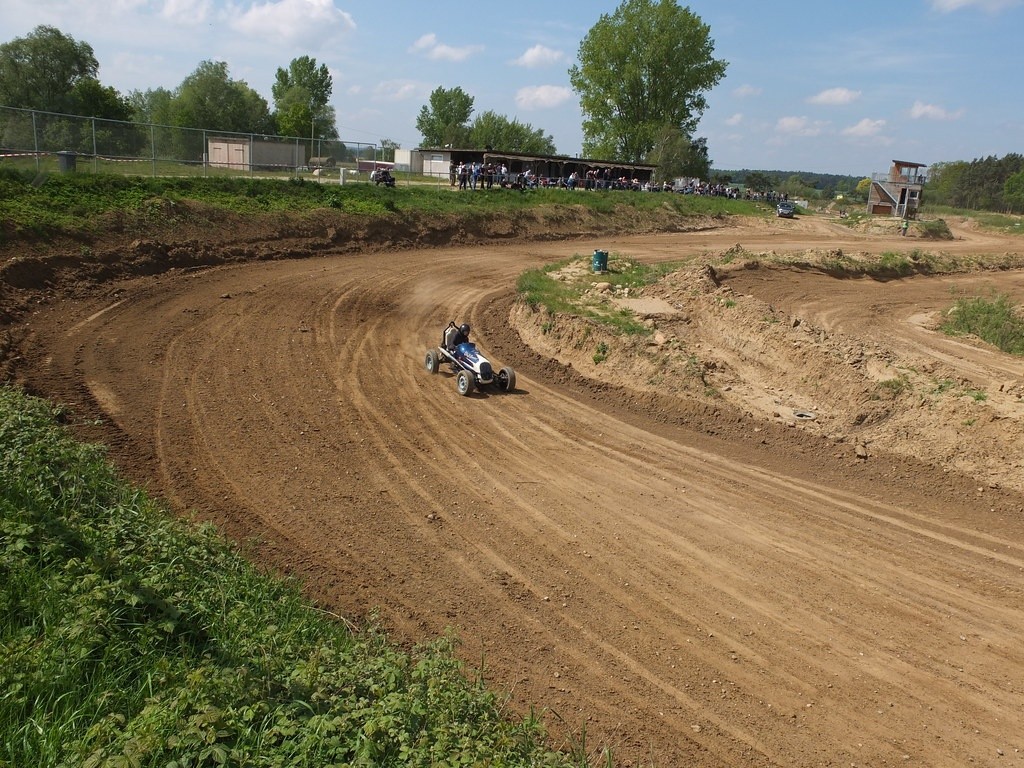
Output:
[593, 250, 608, 271]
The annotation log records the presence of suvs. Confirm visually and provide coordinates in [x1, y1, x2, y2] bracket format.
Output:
[776, 202, 795, 219]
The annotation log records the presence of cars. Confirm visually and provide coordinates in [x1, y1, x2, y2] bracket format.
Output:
[425, 320, 516, 396]
